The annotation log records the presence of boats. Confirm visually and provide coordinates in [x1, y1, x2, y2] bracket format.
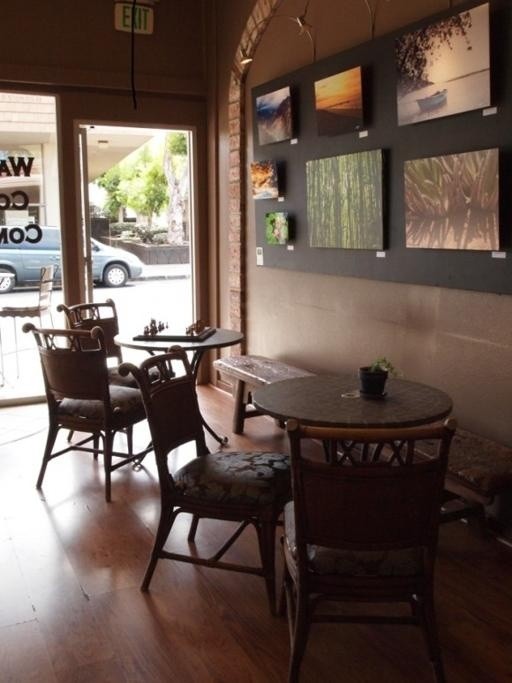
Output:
[416, 88, 448, 112]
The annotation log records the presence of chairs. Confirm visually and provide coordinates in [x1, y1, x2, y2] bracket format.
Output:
[118, 345, 292, 617]
[21, 321, 154, 502]
[57, 298, 137, 458]
[280, 417, 458, 683]
[0, 263, 59, 344]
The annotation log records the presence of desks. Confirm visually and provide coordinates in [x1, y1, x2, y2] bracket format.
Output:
[252, 376, 453, 466]
[112, 327, 245, 465]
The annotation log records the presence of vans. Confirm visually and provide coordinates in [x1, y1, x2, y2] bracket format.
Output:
[0, 224, 143, 294]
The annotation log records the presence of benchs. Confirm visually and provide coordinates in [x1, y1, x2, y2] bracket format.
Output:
[213, 353, 512, 540]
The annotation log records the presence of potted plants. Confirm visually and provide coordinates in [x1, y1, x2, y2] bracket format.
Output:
[358, 356, 400, 398]
